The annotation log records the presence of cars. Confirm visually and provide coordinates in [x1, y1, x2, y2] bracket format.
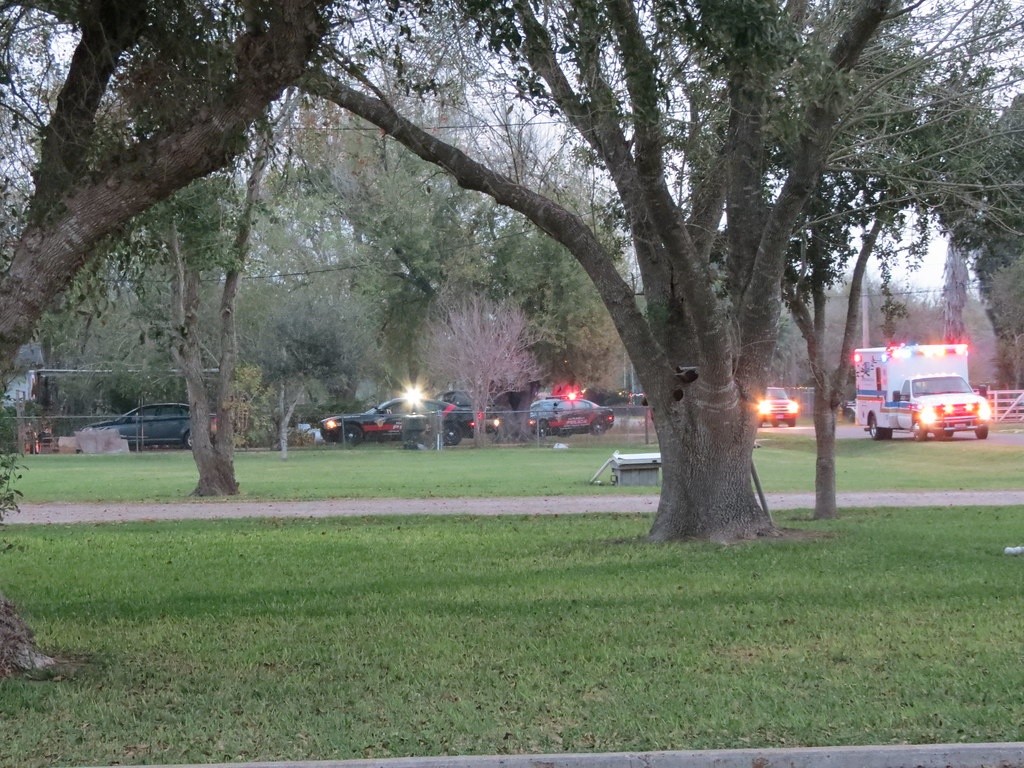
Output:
[81, 403, 219, 451]
[432, 389, 495, 438]
[493, 396, 613, 438]
[756, 386, 797, 427]
[319, 397, 477, 450]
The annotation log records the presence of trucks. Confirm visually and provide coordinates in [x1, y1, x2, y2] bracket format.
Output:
[855, 340, 989, 444]
